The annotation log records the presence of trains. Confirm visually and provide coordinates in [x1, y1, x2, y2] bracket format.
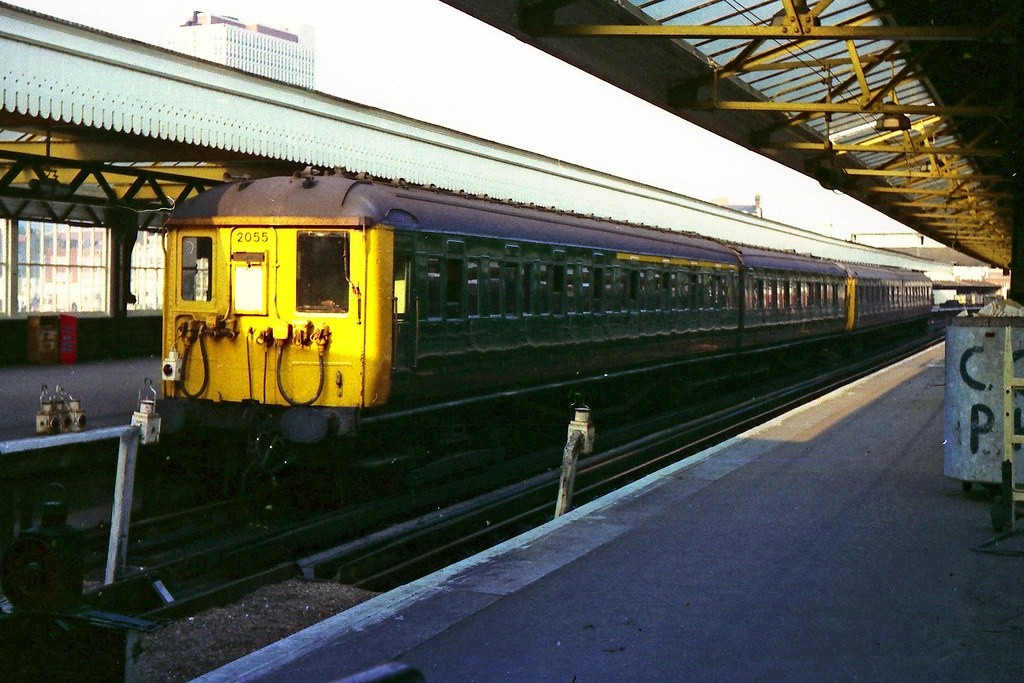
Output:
[155, 168, 938, 508]
